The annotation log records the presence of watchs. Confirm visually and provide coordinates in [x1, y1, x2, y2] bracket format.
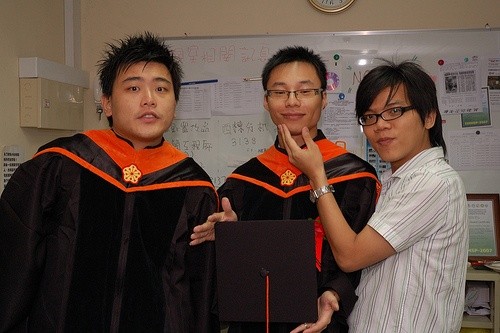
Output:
[309, 184, 335, 203]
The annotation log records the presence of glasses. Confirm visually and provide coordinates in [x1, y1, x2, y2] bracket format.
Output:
[358, 105, 416, 126]
[265, 88, 325, 99]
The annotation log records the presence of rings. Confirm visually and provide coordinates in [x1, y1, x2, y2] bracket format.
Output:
[304, 322, 309, 329]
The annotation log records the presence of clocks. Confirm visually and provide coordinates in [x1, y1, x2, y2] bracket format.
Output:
[308, 0, 358, 15]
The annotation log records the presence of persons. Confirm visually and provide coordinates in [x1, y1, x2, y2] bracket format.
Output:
[189, 46, 382, 333]
[277, 57, 469, 333]
[0, 30, 220, 333]
[445, 76, 456, 92]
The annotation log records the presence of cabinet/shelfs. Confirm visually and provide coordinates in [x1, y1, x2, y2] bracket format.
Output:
[458, 269, 500, 333]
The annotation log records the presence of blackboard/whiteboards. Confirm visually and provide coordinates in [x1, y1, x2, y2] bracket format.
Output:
[154, 27, 500, 194]
